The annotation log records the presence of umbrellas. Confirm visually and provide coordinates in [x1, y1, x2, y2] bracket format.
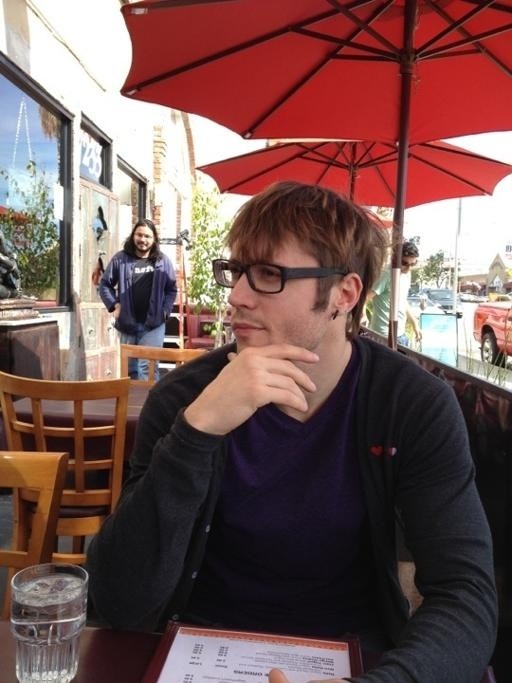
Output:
[195, 139, 511, 209]
[121, 0, 511, 352]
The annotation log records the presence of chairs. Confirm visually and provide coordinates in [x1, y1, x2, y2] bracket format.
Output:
[0, 370, 132, 620]
[120, 343, 209, 374]
[0, 450, 70, 620]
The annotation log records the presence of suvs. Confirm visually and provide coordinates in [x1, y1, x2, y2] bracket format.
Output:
[419, 287, 463, 319]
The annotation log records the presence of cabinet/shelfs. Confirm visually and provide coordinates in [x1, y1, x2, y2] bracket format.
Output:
[0, 319, 61, 399]
[185, 305, 234, 349]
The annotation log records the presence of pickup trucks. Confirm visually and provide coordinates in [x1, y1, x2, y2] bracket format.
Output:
[475, 295, 512, 364]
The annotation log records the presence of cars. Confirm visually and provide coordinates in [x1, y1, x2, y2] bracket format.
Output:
[459, 290, 509, 302]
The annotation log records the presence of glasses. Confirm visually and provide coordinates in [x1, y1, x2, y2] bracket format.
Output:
[212, 258, 350, 291]
[400, 259, 418, 267]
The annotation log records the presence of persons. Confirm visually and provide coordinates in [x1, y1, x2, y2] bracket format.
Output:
[99, 218, 176, 382]
[359, 241, 422, 346]
[84, 179, 498, 682]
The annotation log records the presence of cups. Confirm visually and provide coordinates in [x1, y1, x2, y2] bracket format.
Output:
[11, 564, 89, 683]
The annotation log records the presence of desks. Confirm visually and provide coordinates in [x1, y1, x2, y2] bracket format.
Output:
[1, 621, 364, 683]
[1, 390, 150, 486]
